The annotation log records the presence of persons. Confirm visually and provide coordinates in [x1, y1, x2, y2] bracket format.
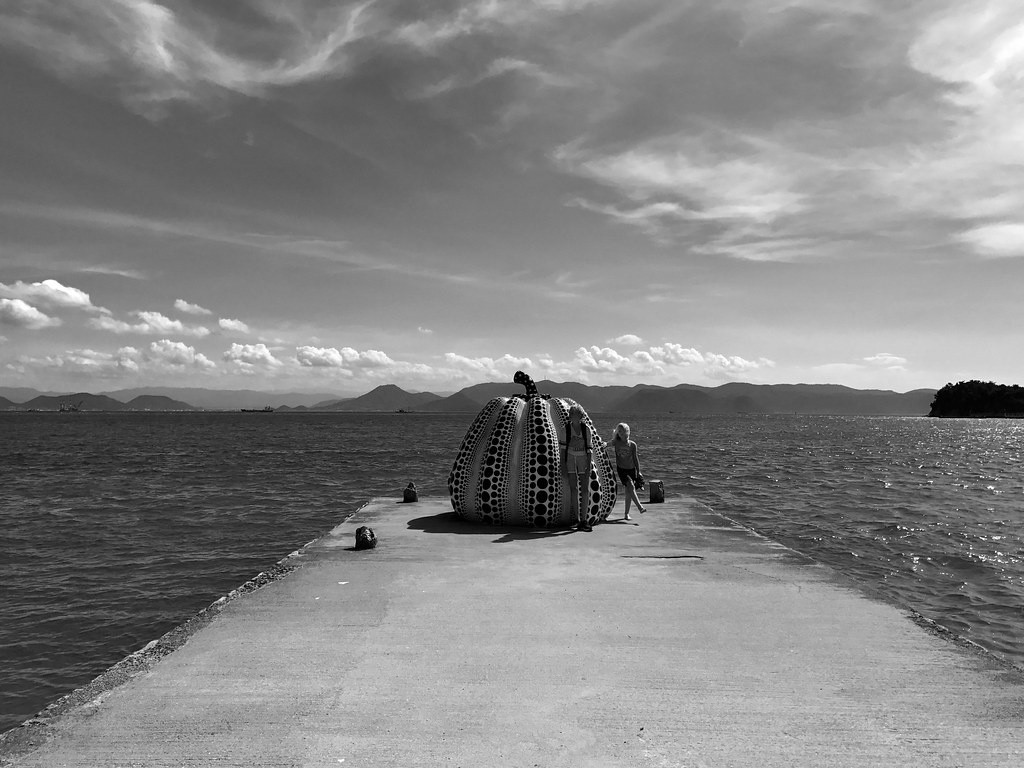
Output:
[560, 405, 594, 532]
[603, 423, 646, 521]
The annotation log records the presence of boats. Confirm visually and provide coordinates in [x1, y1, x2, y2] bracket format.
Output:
[57, 399, 83, 412]
[240, 404, 274, 412]
[395, 409, 415, 414]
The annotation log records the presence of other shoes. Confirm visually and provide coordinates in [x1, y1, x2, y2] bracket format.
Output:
[579, 520, 592, 532]
[570, 519, 580, 530]
[634, 477, 645, 491]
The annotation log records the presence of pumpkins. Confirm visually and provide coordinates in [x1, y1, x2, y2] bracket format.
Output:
[447, 371, 617, 527]
